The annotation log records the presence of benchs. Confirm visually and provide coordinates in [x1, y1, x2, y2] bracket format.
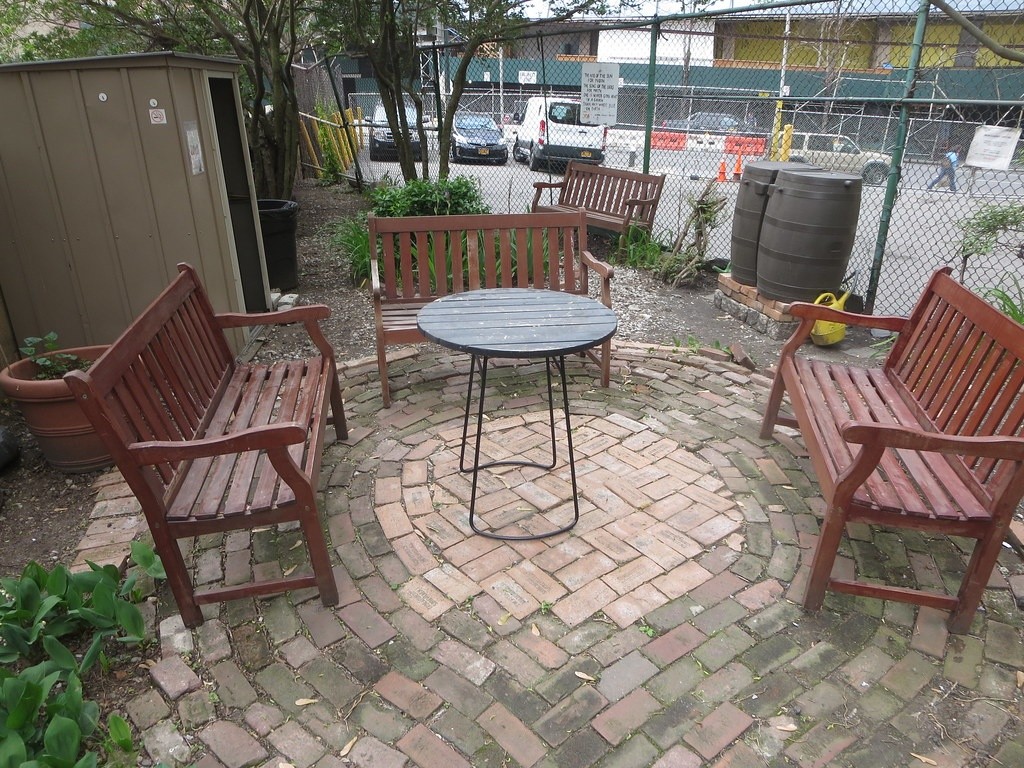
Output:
[368, 206, 614, 409]
[531, 158, 666, 262]
[62, 262, 349, 629]
[759, 265, 1024, 634]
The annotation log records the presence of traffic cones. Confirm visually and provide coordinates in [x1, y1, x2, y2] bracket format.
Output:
[717, 153, 727, 181]
[733, 150, 742, 182]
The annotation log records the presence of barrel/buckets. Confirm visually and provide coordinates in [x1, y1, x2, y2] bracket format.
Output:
[756, 168, 863, 304]
[729, 161, 823, 286]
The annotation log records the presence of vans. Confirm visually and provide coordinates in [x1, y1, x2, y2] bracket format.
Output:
[512, 97, 607, 171]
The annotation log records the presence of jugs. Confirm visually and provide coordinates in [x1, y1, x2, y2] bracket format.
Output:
[808, 290, 852, 345]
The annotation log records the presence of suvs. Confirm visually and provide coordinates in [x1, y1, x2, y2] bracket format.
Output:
[365, 103, 429, 161]
[777, 131, 891, 188]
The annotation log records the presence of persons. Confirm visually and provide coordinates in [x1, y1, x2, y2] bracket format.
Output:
[832, 139, 833, 151]
[927, 145, 961, 191]
[497, 114, 511, 132]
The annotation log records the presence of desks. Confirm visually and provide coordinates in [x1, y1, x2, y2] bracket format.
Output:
[417, 287, 617, 540]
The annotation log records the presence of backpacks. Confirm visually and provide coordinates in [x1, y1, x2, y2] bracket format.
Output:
[940, 152, 957, 170]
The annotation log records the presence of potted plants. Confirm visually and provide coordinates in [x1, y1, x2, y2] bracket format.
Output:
[0, 331, 116, 473]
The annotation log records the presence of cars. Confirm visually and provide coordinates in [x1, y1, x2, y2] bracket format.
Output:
[449, 110, 508, 163]
[663, 112, 771, 138]
